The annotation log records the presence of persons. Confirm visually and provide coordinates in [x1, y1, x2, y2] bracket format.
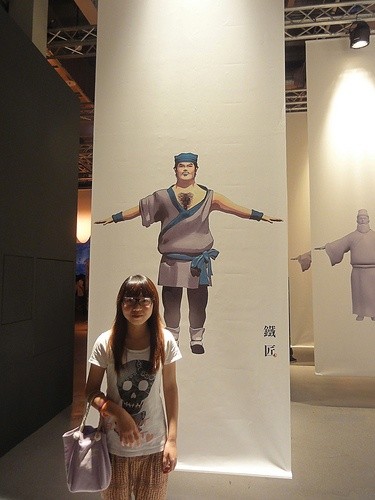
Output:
[84, 275, 183, 500]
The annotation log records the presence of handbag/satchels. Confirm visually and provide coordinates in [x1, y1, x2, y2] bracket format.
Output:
[62, 391, 112, 492]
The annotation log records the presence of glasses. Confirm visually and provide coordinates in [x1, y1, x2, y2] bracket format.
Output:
[121, 296, 154, 308]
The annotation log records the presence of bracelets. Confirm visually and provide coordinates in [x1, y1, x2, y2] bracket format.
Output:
[99, 396, 113, 420]
